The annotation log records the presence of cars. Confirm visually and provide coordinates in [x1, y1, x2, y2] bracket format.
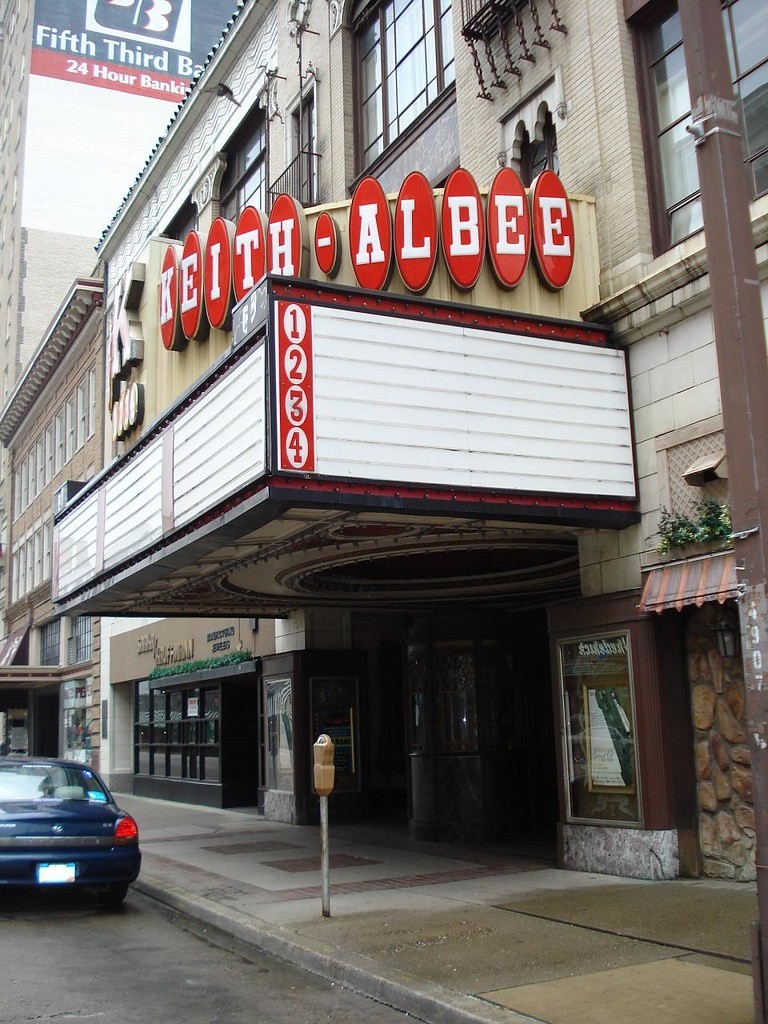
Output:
[0, 753, 140, 909]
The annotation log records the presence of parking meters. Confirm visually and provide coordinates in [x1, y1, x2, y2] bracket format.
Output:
[314, 733, 337, 915]
